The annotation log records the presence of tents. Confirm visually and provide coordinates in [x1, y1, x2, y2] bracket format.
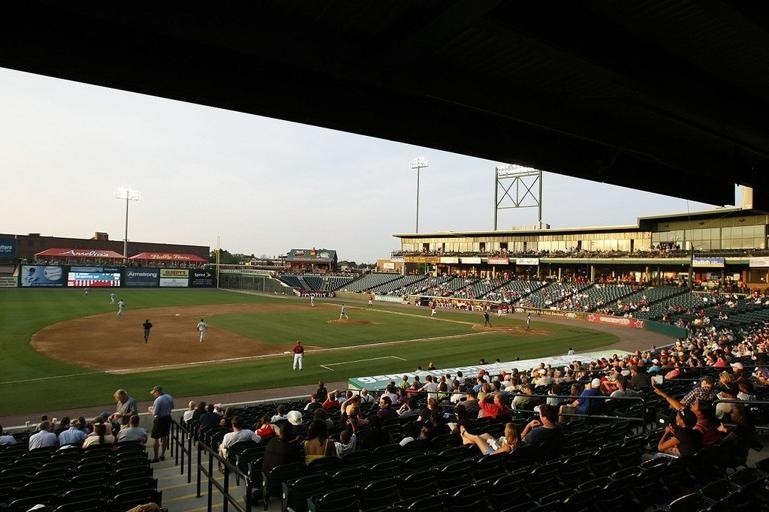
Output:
[35, 249, 127, 265]
[128, 251, 208, 268]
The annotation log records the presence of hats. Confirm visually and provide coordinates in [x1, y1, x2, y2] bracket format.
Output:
[721, 382, 739, 395]
[476, 361, 546, 382]
[150, 385, 162, 395]
[730, 361, 743, 370]
[70, 419, 81, 426]
[286, 410, 303, 426]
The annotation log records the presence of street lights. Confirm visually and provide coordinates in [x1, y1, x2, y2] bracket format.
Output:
[408, 154, 430, 234]
[115, 190, 139, 266]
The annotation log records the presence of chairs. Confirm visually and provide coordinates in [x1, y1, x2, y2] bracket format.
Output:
[213, 247, 769, 346]
[1, 346, 769, 510]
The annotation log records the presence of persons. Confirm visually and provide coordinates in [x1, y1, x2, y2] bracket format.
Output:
[311, 294, 314, 306]
[2, 327, 769, 512]
[368, 295, 373, 307]
[197, 319, 206, 342]
[143, 320, 151, 342]
[23, 268, 38, 285]
[110, 292, 115, 304]
[340, 304, 348, 320]
[117, 299, 124, 315]
[397, 241, 769, 330]
